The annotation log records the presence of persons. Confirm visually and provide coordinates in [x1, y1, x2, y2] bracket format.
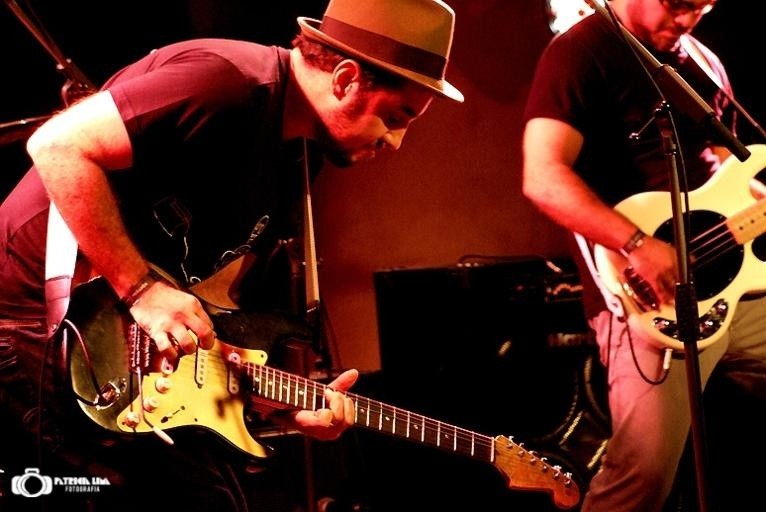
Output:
[2, 1, 468, 511]
[517, 1, 766, 512]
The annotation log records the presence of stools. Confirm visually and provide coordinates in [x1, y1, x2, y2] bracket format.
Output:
[534, 331, 608, 446]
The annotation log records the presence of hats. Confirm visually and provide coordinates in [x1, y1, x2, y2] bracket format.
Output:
[297, 0, 465, 104]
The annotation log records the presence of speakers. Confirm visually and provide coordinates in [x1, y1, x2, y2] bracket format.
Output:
[374, 255, 545, 436]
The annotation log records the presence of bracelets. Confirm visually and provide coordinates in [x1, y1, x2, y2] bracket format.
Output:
[109, 268, 155, 308]
[613, 223, 647, 255]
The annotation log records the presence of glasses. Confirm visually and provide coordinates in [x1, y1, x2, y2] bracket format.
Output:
[661, 0, 716, 17]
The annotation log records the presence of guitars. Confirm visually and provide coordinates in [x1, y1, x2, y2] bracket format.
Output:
[596, 142, 765, 353]
[71, 262, 580, 508]
